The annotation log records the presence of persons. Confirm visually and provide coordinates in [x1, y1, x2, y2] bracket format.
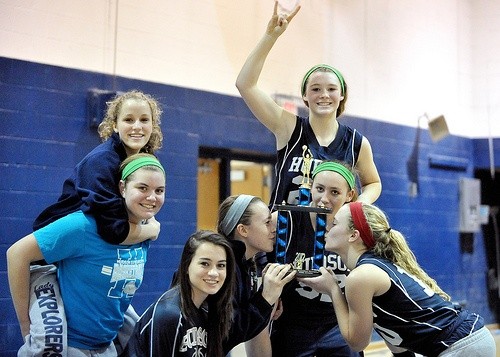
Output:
[119, 229, 238, 357]
[6, 89, 166, 357]
[216, 1, 383, 357]
[295, 201, 497, 357]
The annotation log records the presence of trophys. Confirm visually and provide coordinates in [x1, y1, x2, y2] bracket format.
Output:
[298, 144, 314, 208]
